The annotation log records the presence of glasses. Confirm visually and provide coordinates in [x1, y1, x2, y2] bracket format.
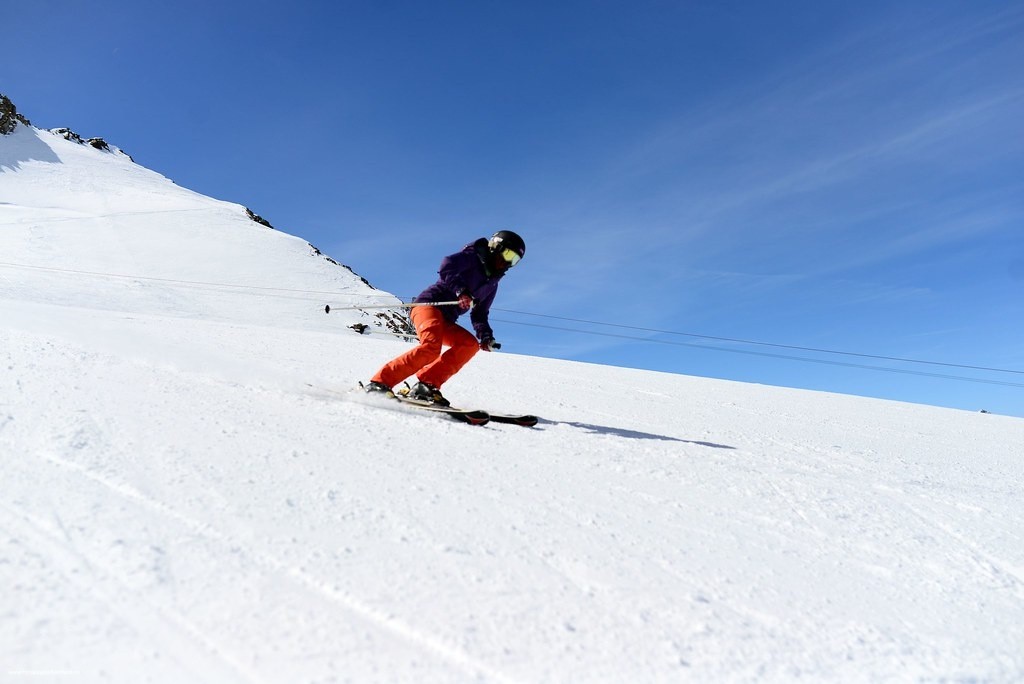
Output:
[502, 248, 521, 267]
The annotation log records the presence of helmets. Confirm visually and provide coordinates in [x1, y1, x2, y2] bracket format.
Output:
[487, 230, 525, 275]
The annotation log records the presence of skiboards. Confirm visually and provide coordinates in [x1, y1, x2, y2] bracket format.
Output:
[345, 382, 539, 427]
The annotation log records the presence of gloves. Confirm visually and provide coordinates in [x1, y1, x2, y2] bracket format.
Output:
[480, 336, 496, 352]
[459, 289, 477, 309]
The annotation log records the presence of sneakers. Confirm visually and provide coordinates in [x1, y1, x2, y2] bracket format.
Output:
[364, 382, 402, 403]
[406, 381, 450, 408]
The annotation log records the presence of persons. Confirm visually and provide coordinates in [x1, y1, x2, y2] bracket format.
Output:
[364, 229, 527, 408]
